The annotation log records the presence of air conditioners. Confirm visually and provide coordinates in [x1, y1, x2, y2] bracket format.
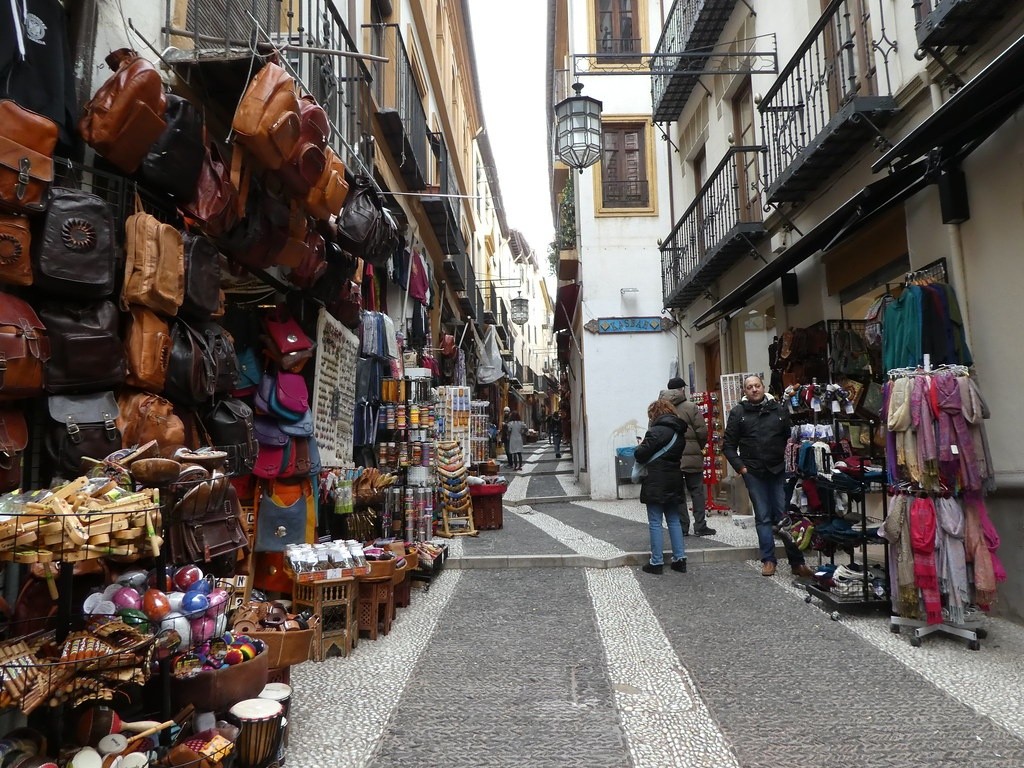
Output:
[270, 32, 344, 157]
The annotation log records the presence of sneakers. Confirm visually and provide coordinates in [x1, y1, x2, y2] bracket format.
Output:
[762, 562, 775, 575]
[791, 564, 815, 576]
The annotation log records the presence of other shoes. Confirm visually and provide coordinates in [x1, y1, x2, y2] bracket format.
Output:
[669, 555, 688, 571]
[513, 467, 522, 471]
[505, 464, 513, 468]
[643, 564, 665, 574]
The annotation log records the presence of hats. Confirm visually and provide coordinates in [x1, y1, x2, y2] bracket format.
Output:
[668, 378, 688, 389]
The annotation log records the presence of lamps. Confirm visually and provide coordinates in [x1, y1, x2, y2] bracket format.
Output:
[510, 289, 528, 326]
[620, 288, 640, 294]
[552, 29, 779, 177]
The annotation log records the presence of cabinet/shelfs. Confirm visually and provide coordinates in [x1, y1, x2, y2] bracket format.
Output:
[803, 405, 892, 621]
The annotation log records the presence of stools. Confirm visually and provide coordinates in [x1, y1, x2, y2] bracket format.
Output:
[357, 574, 394, 642]
[267, 665, 291, 749]
[293, 575, 356, 662]
[472, 493, 503, 530]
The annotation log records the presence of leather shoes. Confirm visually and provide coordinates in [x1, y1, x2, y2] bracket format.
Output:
[682, 532, 689, 538]
[695, 525, 716, 537]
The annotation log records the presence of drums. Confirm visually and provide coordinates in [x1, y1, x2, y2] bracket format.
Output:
[258, 682, 293, 748]
[230, 698, 285, 768]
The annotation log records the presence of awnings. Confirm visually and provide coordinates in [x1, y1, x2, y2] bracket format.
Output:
[871, 34, 1024, 174]
[691, 154, 938, 330]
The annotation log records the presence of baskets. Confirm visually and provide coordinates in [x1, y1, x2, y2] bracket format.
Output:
[153, 707, 243, 768]
[0, 462, 236, 722]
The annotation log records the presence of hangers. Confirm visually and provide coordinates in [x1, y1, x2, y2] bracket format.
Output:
[886, 363, 971, 382]
[890, 478, 949, 497]
[903, 268, 938, 292]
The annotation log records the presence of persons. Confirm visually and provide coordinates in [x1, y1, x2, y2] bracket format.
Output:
[552, 411, 562, 458]
[658, 378, 717, 537]
[722, 376, 806, 576]
[500, 411, 528, 471]
[544, 413, 553, 444]
[634, 400, 688, 575]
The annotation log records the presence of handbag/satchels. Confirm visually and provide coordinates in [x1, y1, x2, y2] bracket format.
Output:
[632, 460, 647, 482]
[0, 48, 398, 567]
